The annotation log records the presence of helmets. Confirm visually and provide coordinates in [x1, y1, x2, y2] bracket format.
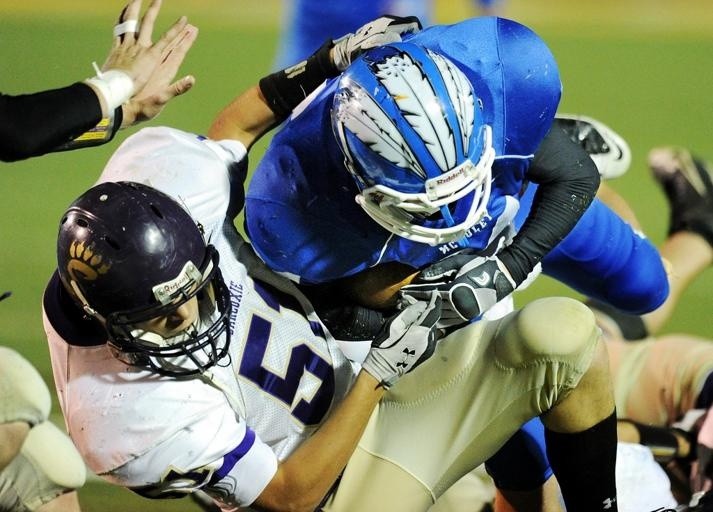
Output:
[56, 180, 231, 377]
[331, 42, 495, 249]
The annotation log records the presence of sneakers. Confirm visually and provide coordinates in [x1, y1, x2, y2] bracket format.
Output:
[554, 115, 632, 181]
[645, 145, 712, 222]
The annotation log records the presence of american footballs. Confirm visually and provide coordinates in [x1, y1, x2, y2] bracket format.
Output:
[350, 261, 422, 310]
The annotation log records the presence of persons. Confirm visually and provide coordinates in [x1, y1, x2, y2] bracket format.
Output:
[39, 0, 711, 512]
[0, 344, 88, 511]
[0, 1, 200, 164]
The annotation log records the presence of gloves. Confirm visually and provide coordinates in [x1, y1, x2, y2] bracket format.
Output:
[397, 253, 517, 329]
[310, 14, 422, 80]
[360, 289, 444, 392]
